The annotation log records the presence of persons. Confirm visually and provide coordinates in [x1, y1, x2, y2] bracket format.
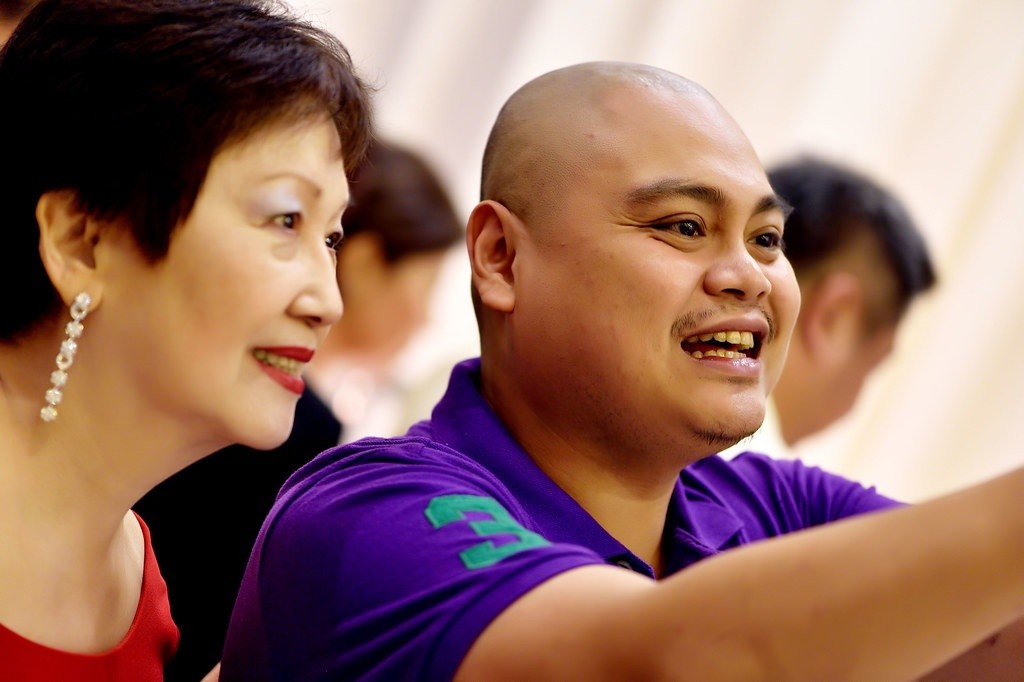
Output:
[710, 154, 936, 462]
[130, 143, 468, 682]
[218, 62, 1024, 682]
[0, 0, 379, 682]
